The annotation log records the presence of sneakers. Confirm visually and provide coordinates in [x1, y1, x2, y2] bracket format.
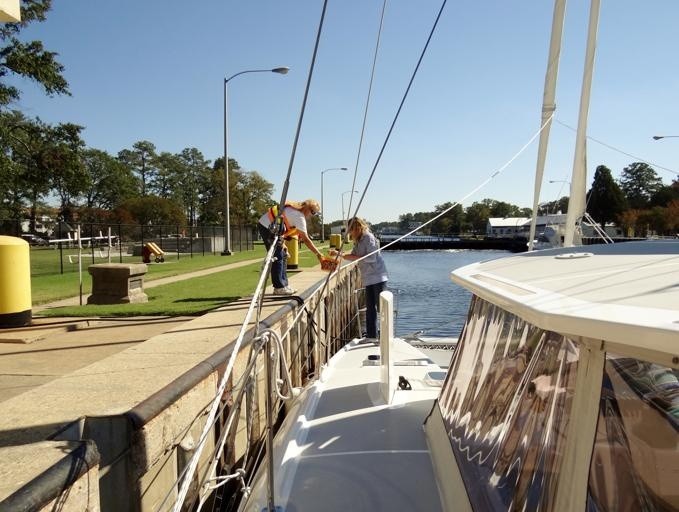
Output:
[359, 337, 378, 344]
[273, 285, 298, 296]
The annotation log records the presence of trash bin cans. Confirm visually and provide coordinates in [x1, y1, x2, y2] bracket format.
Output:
[0, 235, 32, 328]
[285, 235, 298, 269]
[330, 234, 336, 248]
[336, 234, 341, 251]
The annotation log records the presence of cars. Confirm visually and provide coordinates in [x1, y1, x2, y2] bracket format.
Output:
[22, 234, 49, 247]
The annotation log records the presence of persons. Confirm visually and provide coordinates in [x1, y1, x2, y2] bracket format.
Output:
[256, 199, 390, 344]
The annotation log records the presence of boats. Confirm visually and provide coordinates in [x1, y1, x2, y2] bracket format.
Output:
[164, 5, 677, 508]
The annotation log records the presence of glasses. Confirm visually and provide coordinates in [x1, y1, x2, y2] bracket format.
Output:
[310, 209, 316, 216]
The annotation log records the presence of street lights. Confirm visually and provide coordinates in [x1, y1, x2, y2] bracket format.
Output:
[222, 65, 292, 255]
[653, 135, 678, 140]
[341, 190, 359, 226]
[549, 180, 571, 192]
[320, 168, 347, 240]
